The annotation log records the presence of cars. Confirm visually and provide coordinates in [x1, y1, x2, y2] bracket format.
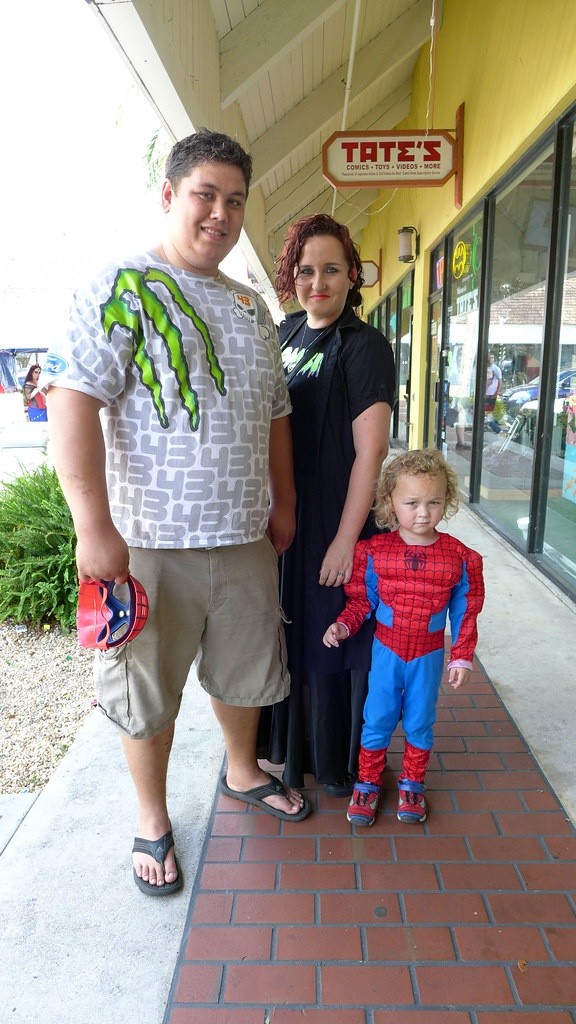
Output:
[501, 366, 576, 432]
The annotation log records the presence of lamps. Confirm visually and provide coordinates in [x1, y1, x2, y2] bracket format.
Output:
[398, 226, 421, 264]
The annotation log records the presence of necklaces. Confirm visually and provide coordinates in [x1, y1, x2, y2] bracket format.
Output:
[299, 323, 328, 351]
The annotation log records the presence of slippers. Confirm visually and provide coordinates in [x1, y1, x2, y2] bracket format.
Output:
[132, 819, 184, 897]
[220, 769, 311, 822]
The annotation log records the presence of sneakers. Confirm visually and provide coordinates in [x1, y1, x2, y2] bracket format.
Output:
[397, 779, 427, 824]
[347, 780, 382, 827]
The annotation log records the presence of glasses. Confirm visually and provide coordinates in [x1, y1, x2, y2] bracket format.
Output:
[34, 371, 41, 374]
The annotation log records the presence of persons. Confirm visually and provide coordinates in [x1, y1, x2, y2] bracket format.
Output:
[322, 451, 485, 828]
[33, 128, 298, 896]
[23, 364, 47, 422]
[445, 348, 503, 450]
[255, 214, 398, 797]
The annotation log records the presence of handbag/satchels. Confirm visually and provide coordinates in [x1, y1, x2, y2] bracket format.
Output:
[28, 407, 48, 422]
[444, 405, 459, 427]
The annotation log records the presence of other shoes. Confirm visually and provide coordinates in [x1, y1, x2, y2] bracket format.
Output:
[456, 442, 472, 450]
[322, 771, 355, 796]
